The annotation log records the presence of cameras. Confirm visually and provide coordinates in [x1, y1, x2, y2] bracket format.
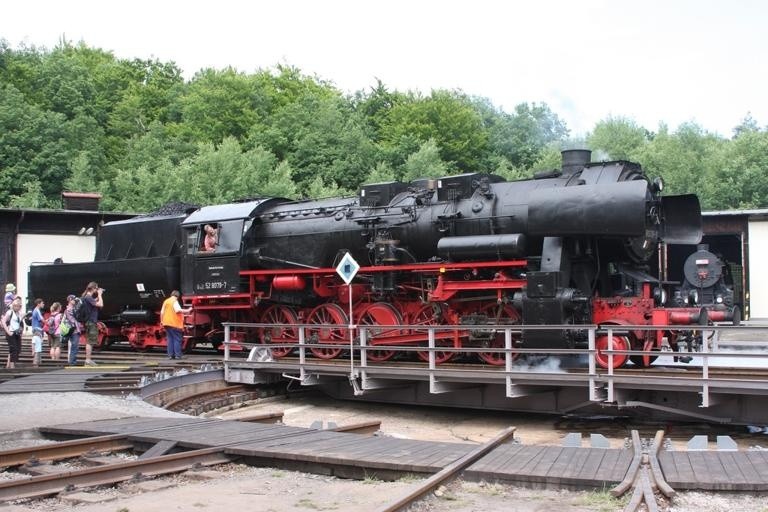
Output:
[102, 289, 106, 293]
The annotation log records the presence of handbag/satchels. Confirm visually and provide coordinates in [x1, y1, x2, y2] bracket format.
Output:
[61, 311, 76, 337]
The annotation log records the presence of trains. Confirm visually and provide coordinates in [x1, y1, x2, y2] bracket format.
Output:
[25, 150, 741, 368]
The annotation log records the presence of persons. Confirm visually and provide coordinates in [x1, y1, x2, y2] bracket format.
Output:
[44, 309, 51, 346]
[2, 299, 24, 368]
[65, 294, 83, 365]
[31, 298, 47, 370]
[160, 290, 193, 360]
[82, 281, 104, 367]
[204, 225, 217, 251]
[4, 284, 17, 307]
[50, 302, 63, 359]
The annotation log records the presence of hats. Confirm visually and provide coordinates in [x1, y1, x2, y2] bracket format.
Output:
[32, 327, 42, 333]
[67, 295, 75, 301]
[5, 283, 16, 291]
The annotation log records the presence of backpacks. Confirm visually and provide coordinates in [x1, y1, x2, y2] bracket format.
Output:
[48, 314, 60, 335]
[0, 310, 13, 329]
[73, 296, 93, 323]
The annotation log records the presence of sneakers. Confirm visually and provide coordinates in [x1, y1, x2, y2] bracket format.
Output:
[85, 360, 97, 367]
